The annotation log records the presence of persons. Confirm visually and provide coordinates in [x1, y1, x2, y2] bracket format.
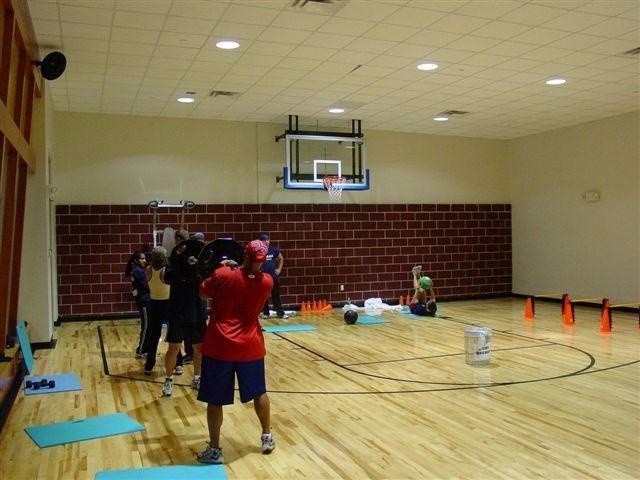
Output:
[197, 240, 276, 463]
[410, 266, 437, 317]
[126, 229, 208, 396]
[257, 235, 289, 319]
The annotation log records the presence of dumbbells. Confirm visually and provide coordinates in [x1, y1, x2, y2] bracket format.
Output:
[26, 379, 55, 389]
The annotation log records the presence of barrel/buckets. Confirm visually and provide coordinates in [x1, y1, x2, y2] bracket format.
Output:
[464, 325, 491, 364]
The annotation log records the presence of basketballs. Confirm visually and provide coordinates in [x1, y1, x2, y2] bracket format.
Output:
[344, 310, 357, 324]
[419, 276, 433, 290]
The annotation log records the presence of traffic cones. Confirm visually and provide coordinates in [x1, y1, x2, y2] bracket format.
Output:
[523, 297, 533, 318]
[598, 308, 611, 332]
[398, 295, 404, 306]
[564, 299, 573, 325]
[406, 295, 411, 305]
[300, 299, 332, 312]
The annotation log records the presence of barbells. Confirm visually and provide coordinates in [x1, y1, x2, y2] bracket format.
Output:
[171, 239, 244, 282]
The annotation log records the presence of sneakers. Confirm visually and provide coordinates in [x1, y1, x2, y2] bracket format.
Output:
[191, 377, 204, 390]
[183, 354, 193, 365]
[278, 314, 288, 319]
[196, 447, 224, 465]
[175, 366, 184, 374]
[163, 382, 173, 396]
[261, 432, 275, 453]
[412, 266, 422, 275]
[136, 353, 147, 359]
